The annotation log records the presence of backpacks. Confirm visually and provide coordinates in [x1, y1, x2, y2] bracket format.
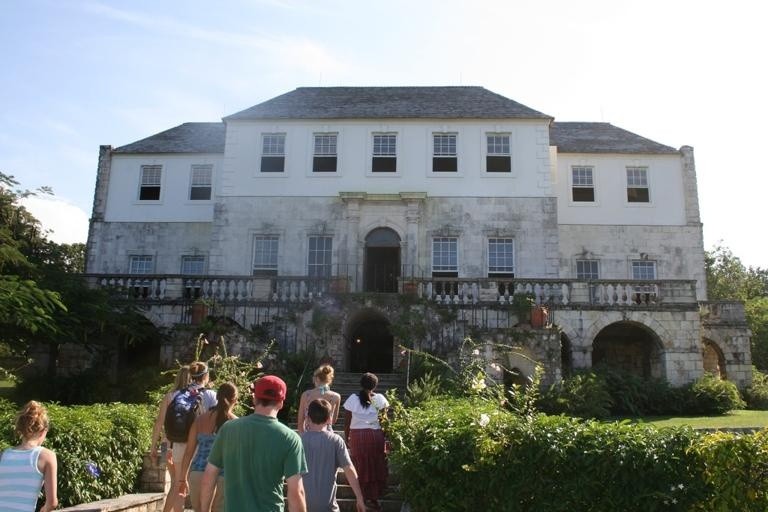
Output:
[164, 388, 210, 442]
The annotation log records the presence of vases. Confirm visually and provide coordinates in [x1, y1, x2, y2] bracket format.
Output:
[403, 283, 418, 294]
[333, 279, 351, 294]
[531, 307, 548, 329]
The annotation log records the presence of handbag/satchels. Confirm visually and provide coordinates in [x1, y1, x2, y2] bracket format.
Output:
[384, 441, 390, 454]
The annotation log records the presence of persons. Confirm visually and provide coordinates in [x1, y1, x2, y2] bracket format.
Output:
[148, 360, 391, 511]
[0, 399, 59, 512]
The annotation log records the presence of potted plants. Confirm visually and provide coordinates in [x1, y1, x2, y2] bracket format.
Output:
[191, 294, 220, 324]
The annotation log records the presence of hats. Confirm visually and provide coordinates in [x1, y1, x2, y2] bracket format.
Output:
[255, 375, 287, 400]
